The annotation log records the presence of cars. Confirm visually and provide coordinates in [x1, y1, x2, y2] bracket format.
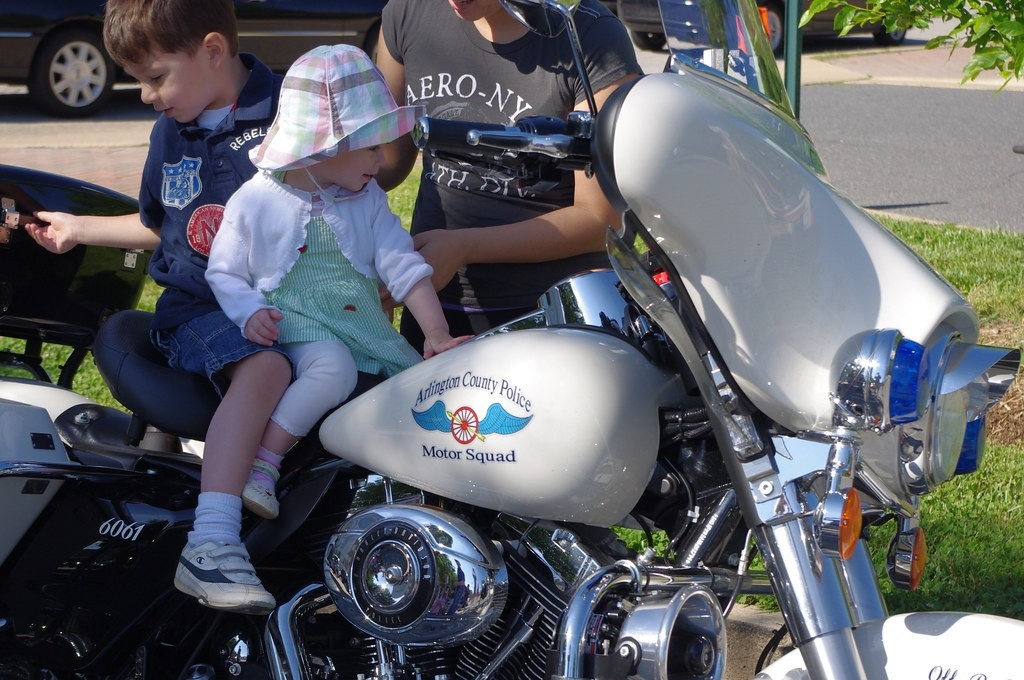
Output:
[616, 0, 916, 58]
[0, 1, 386, 118]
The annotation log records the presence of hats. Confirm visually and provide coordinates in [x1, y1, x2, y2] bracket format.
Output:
[247, 43, 423, 170]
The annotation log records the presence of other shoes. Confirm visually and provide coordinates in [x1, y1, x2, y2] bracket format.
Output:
[239, 456, 280, 519]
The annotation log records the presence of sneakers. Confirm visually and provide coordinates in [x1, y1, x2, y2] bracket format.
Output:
[173, 537, 277, 614]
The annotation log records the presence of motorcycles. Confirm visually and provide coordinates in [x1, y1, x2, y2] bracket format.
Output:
[1, 1, 1021, 679]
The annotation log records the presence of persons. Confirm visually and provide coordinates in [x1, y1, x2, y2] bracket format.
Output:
[376, 1, 644, 360]
[204, 44, 476, 519]
[22, 0, 284, 610]
